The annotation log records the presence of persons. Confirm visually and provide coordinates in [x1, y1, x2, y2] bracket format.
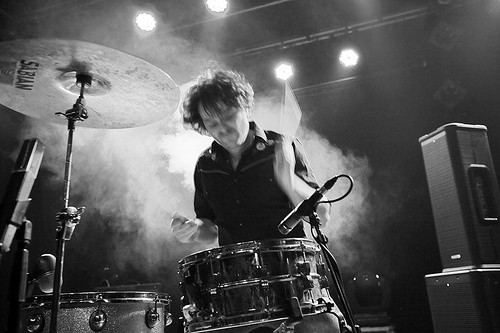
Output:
[171, 70, 340, 333]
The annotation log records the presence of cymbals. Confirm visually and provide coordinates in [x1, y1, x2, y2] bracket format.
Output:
[0, 41, 180, 128]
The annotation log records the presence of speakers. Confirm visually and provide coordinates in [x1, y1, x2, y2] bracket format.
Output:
[424, 269, 500, 333]
[418, 123, 500, 272]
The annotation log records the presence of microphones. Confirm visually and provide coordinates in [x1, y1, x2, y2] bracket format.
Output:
[277, 175, 338, 235]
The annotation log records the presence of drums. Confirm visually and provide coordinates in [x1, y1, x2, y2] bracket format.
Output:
[18, 290, 173, 333]
[176, 238, 333, 333]
